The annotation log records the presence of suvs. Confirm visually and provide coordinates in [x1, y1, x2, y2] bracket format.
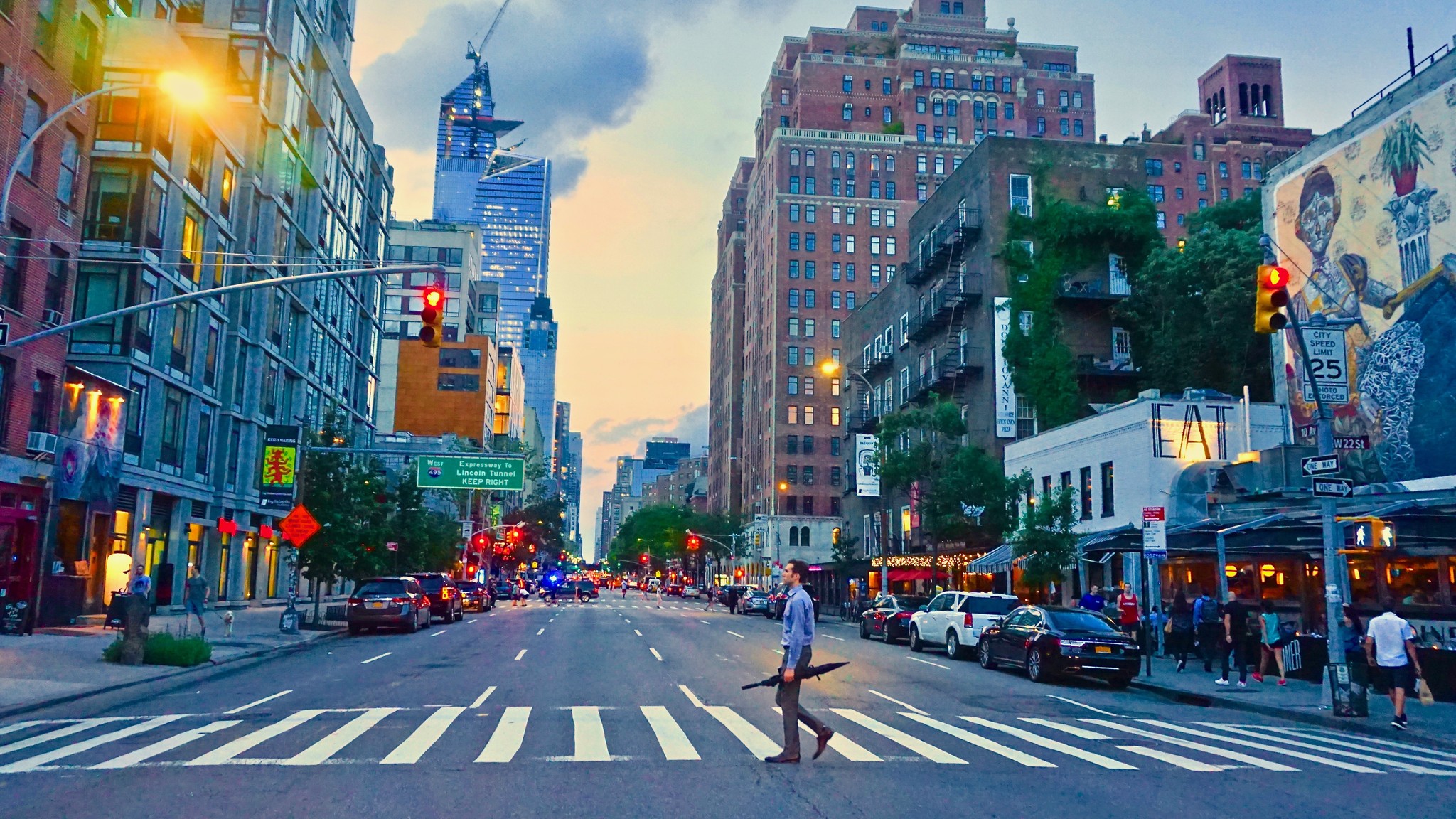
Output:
[908, 589, 1020, 661]
[715, 582, 821, 623]
[542, 581, 600, 603]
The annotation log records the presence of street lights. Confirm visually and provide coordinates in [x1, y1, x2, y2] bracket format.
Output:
[774, 483, 788, 566]
[727, 456, 763, 515]
[821, 358, 889, 598]
[638, 539, 650, 575]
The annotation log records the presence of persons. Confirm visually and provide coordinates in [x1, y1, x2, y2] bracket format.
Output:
[183, 565, 210, 637]
[126, 565, 152, 600]
[1080, 582, 1422, 730]
[929, 582, 943, 598]
[487, 578, 778, 616]
[763, 559, 834, 765]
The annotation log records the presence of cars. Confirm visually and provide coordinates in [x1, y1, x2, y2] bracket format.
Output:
[455, 578, 536, 612]
[858, 594, 934, 645]
[597, 574, 664, 594]
[977, 604, 1142, 690]
[681, 585, 700, 598]
[346, 576, 432, 638]
[404, 571, 465, 625]
[667, 584, 683, 597]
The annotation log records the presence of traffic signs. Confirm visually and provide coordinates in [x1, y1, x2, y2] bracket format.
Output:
[1300, 453, 1341, 478]
[1311, 476, 1355, 499]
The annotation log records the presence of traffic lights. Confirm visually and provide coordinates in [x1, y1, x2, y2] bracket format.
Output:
[755, 534, 759, 545]
[1379, 520, 1397, 551]
[686, 539, 699, 551]
[1254, 264, 1290, 335]
[418, 288, 446, 349]
[506, 530, 520, 544]
[1353, 519, 1383, 551]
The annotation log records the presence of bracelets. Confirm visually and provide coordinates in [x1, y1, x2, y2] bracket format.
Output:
[145, 591, 148, 593]
[1226, 634, 1230, 636]
[205, 597, 208, 600]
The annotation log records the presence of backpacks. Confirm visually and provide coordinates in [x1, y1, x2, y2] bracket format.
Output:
[1200, 598, 1219, 624]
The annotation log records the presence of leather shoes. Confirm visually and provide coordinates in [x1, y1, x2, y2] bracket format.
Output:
[813, 726, 834, 759]
[765, 750, 801, 763]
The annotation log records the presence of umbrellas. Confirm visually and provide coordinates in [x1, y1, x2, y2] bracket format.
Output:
[878, 569, 951, 598]
[741, 661, 851, 690]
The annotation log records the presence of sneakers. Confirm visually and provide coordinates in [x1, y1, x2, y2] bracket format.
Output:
[1400, 714, 1408, 724]
[1391, 715, 1408, 730]
[1215, 678, 1229, 685]
[1236, 681, 1247, 687]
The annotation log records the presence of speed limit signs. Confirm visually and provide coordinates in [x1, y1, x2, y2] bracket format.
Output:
[1300, 326, 1350, 385]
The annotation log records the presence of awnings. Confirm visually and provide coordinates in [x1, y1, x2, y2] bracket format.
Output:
[1017, 542, 1087, 570]
[965, 542, 1035, 573]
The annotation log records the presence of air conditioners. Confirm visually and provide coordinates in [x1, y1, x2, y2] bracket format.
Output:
[26, 431, 58, 454]
[42, 308, 64, 327]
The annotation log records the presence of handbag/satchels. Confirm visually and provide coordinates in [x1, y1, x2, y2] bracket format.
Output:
[1164, 616, 1173, 633]
[1415, 673, 1434, 705]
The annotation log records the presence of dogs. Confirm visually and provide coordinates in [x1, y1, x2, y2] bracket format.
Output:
[223, 611, 234, 636]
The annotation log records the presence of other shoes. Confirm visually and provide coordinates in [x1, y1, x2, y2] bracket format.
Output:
[522, 605, 527, 606]
[1180, 669, 1183, 673]
[491, 605, 496, 608]
[1278, 680, 1286, 685]
[1176, 661, 1182, 672]
[1252, 673, 1263, 683]
[1204, 662, 1212, 672]
[201, 627, 205, 635]
[657, 606, 661, 608]
